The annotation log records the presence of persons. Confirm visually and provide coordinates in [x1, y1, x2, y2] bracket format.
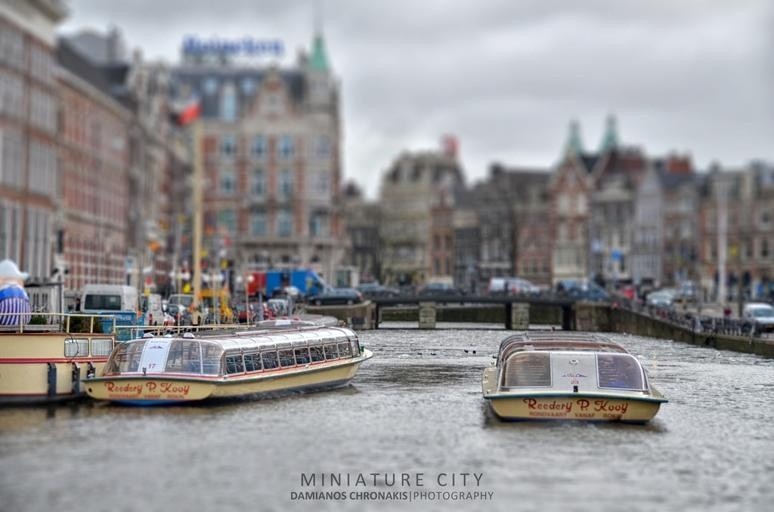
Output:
[370, 272, 765, 339]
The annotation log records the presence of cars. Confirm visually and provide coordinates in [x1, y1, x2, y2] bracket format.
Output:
[419, 283, 454, 296]
[556, 278, 610, 302]
[308, 285, 399, 305]
[743, 302, 774, 332]
[80, 284, 301, 322]
[645, 279, 695, 308]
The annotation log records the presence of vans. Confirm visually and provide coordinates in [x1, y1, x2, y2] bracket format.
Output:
[488, 277, 541, 296]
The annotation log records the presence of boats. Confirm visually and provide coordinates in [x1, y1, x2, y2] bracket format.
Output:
[482, 331, 669, 420]
[0, 313, 373, 407]
